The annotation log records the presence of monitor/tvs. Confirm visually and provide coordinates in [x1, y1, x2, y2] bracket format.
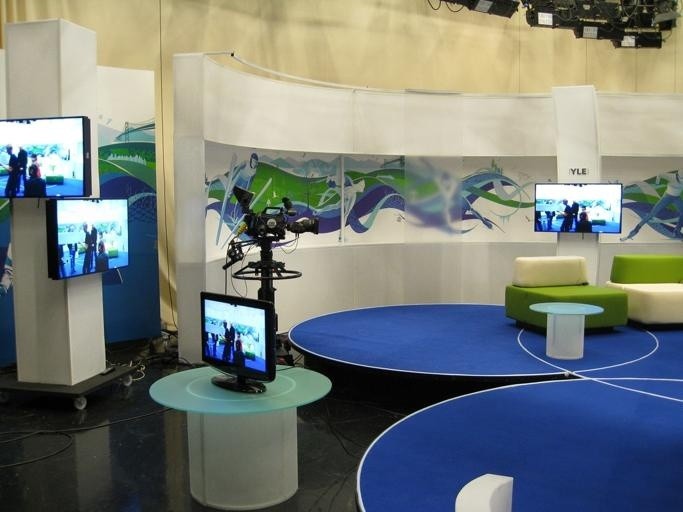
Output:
[534, 182, 623, 234]
[200, 291, 276, 393]
[0, 115, 91, 198]
[46, 198, 130, 280]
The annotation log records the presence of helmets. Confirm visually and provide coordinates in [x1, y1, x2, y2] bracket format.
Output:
[250, 153, 258, 168]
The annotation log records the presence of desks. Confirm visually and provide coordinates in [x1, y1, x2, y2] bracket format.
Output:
[148, 364, 333, 511]
[528, 302, 605, 360]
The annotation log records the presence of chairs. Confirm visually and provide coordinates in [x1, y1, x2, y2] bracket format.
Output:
[606, 254, 683, 330]
[504, 256, 628, 335]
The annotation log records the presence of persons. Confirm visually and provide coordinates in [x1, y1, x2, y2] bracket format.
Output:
[0, 144, 47, 197]
[57, 223, 108, 277]
[204, 319, 245, 368]
[537, 200, 592, 231]
